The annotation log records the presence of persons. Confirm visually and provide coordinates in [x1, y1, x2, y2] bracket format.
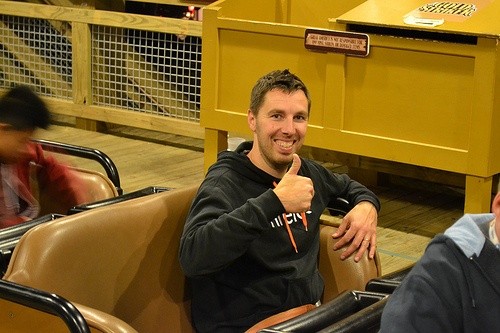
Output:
[380, 173, 500, 333]
[178, 68, 381, 333]
[0, 86, 84, 230]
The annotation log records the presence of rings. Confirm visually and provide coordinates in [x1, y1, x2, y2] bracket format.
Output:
[363, 239, 370, 241]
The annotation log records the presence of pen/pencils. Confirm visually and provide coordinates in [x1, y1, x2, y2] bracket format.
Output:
[416, 22, 433, 25]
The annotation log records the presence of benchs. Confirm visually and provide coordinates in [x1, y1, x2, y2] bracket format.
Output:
[0, 138, 500, 333]
